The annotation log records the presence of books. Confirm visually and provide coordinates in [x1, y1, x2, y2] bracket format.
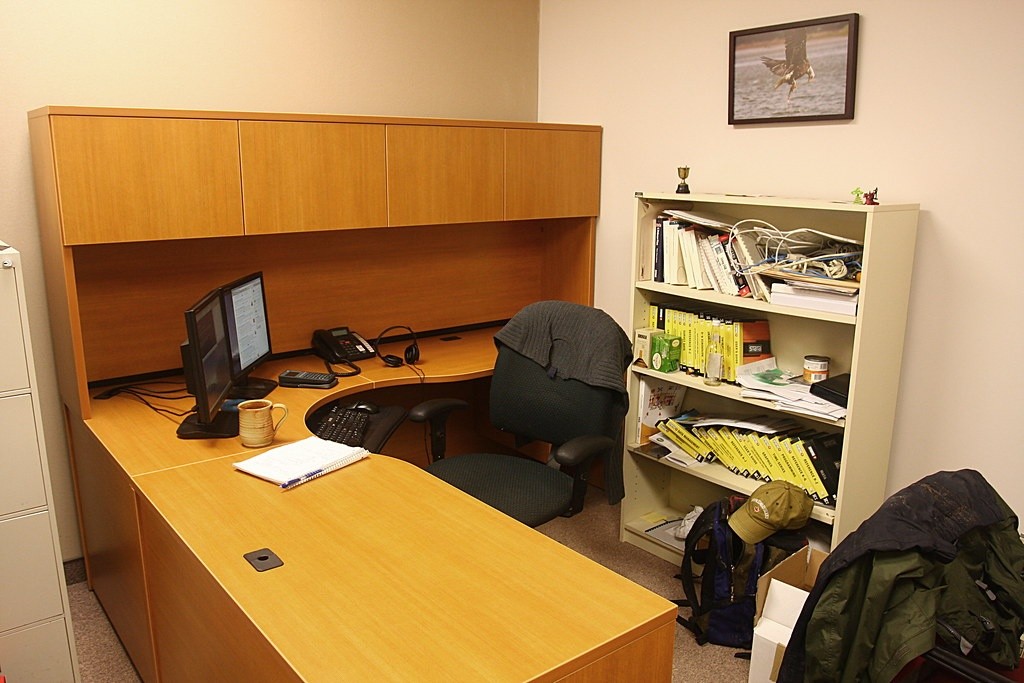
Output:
[647, 300, 773, 388]
[634, 375, 844, 510]
[647, 213, 861, 317]
[233, 435, 370, 490]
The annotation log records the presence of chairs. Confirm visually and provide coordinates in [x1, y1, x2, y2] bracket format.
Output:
[408, 299, 626, 526]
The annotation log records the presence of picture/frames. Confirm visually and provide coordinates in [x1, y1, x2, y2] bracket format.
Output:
[729, 13, 859, 124]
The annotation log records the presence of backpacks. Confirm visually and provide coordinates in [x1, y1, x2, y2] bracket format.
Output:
[669, 495, 810, 660]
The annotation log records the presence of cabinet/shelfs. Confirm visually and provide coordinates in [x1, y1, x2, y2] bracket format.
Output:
[619, 191, 921, 588]
[29, 108, 602, 249]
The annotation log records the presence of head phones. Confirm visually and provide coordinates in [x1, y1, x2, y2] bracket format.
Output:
[375, 326, 419, 367]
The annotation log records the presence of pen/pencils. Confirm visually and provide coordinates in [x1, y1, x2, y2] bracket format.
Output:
[281, 467, 322, 487]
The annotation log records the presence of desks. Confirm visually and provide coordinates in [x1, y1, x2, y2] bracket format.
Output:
[61, 328, 677, 683]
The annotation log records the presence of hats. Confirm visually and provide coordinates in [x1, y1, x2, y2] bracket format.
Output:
[728, 480, 814, 545]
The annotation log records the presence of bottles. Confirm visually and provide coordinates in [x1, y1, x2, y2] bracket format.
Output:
[803, 355, 830, 384]
[704, 321, 724, 386]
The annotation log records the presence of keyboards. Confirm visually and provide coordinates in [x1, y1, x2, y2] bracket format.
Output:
[316, 406, 369, 448]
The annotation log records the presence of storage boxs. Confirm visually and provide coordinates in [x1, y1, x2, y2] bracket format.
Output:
[749, 544, 832, 683]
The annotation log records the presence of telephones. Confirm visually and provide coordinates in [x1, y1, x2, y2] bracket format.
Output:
[312, 327, 375, 364]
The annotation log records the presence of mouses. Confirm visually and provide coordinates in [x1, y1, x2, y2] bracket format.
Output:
[346, 402, 379, 414]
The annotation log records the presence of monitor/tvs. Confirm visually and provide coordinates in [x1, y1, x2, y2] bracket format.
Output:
[176, 268, 279, 440]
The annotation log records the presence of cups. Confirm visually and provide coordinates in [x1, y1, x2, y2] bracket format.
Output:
[237, 400, 288, 447]
